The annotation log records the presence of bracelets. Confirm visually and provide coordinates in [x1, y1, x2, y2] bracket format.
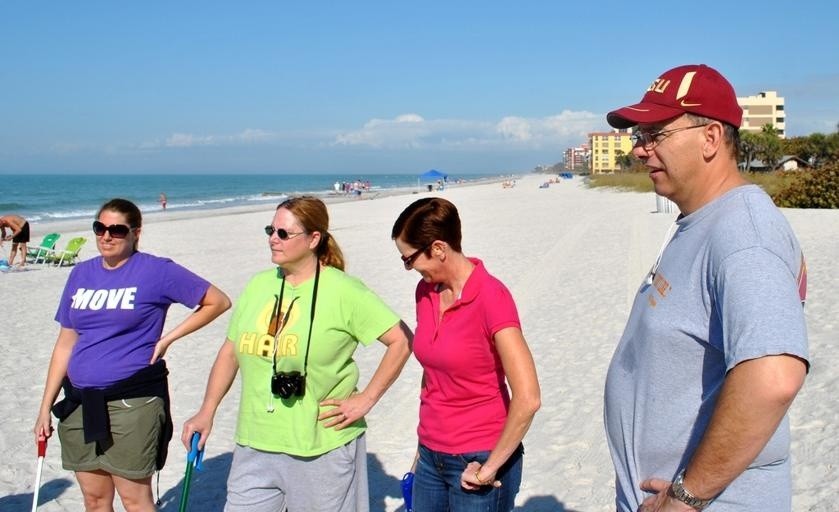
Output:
[670, 468, 725, 511]
[475, 462, 496, 486]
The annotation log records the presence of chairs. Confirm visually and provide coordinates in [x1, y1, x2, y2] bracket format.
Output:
[19, 233, 87, 268]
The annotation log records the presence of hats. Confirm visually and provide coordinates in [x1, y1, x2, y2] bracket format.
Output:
[606, 63, 743, 129]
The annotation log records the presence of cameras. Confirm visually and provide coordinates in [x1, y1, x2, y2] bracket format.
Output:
[271, 372, 305, 400]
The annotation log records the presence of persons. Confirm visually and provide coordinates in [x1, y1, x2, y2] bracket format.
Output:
[33, 198, 233, 512]
[334, 179, 370, 195]
[603, 63, 812, 511]
[179, 197, 413, 512]
[0, 214, 30, 267]
[389, 197, 542, 512]
[160, 192, 167, 210]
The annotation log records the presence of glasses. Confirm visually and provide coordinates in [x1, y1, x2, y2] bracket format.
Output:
[264, 225, 308, 240]
[92, 220, 136, 240]
[627, 123, 711, 153]
[401, 241, 434, 271]
